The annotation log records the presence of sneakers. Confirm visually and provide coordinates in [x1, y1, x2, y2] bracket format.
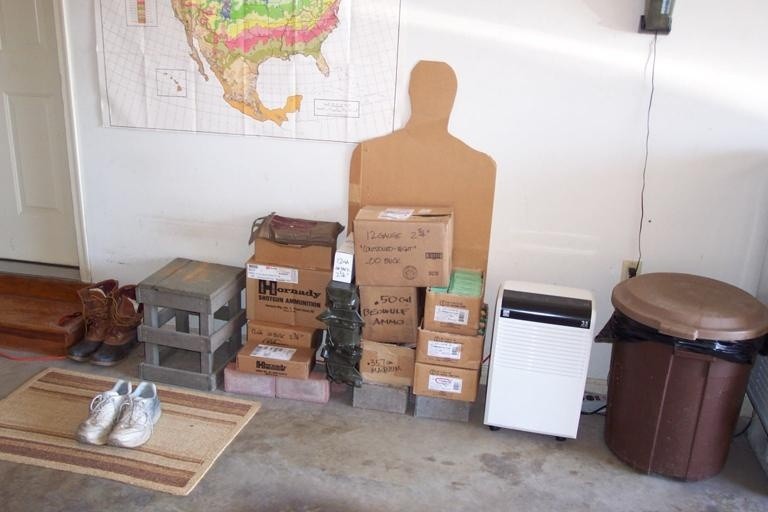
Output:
[107, 379, 163, 448]
[74, 380, 133, 446]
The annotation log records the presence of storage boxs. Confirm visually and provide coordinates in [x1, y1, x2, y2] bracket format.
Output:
[245, 318, 317, 349]
[412, 361, 479, 403]
[243, 254, 333, 331]
[358, 285, 419, 344]
[248, 212, 344, 272]
[353, 204, 456, 288]
[414, 304, 489, 371]
[238, 344, 316, 380]
[357, 338, 416, 389]
[424, 269, 487, 337]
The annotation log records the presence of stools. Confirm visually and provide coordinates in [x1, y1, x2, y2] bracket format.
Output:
[132, 250, 245, 394]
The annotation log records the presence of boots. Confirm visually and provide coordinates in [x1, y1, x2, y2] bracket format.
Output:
[88, 284, 144, 367]
[66, 278, 118, 363]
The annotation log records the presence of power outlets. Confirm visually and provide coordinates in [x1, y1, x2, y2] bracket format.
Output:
[619, 260, 646, 286]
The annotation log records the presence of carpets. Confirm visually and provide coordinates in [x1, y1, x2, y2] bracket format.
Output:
[0, 361, 264, 500]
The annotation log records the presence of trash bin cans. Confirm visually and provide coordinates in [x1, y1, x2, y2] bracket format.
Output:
[594, 273, 768, 482]
